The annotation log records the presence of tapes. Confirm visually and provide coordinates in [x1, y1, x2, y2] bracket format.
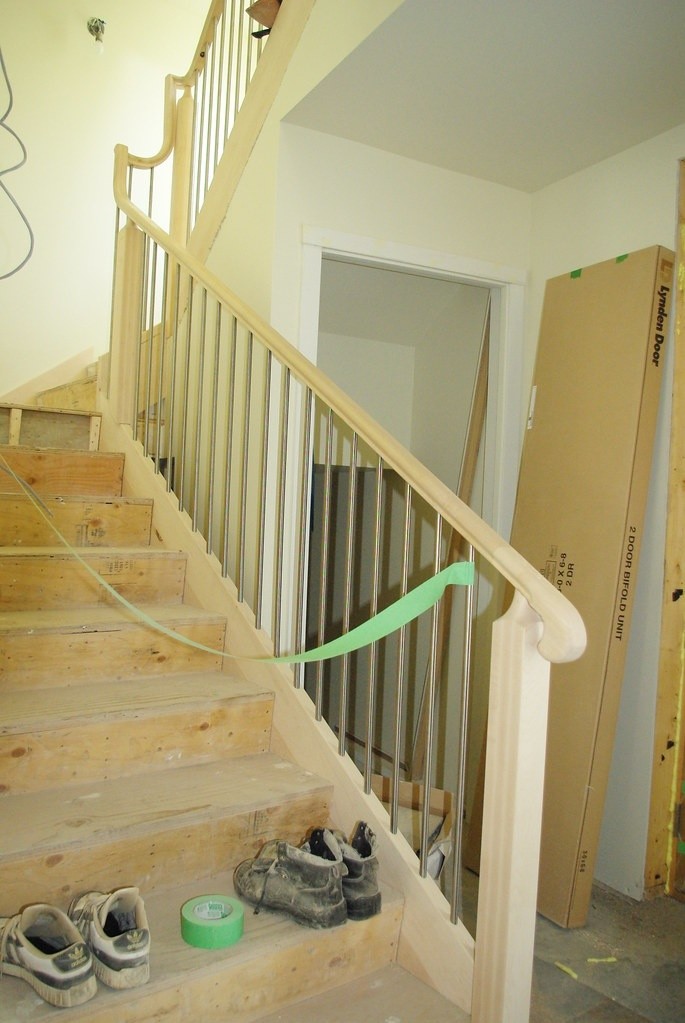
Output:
[180, 895, 245, 949]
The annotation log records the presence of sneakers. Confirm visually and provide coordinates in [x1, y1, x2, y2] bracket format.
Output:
[295, 820, 382, 921]
[232, 828, 346, 929]
[66, 885, 150, 989]
[0, 904, 98, 1008]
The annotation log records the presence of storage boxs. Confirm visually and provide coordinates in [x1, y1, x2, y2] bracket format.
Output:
[456, 245, 674, 924]
